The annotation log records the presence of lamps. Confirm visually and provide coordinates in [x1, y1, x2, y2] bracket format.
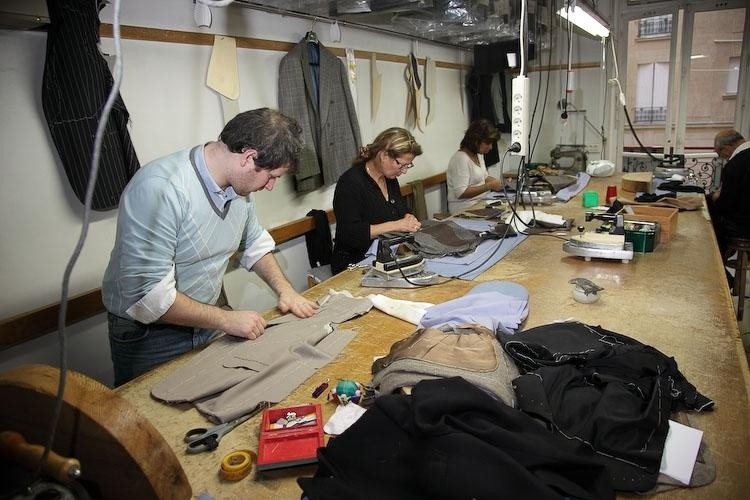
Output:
[555, 0, 611, 39]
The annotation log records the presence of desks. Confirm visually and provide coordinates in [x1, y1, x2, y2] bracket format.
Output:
[111, 174, 750, 498]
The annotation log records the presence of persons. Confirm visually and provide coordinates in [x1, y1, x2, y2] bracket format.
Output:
[99, 107, 321, 388]
[330, 127, 423, 277]
[703, 130, 750, 296]
[447, 118, 501, 216]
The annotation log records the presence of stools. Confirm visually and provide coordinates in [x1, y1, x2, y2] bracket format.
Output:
[306, 263, 332, 288]
[726, 239, 750, 321]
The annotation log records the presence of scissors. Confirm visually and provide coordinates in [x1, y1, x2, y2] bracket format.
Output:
[184, 405, 267, 454]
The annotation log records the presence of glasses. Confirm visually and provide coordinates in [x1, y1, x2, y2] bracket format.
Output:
[396, 159, 414, 169]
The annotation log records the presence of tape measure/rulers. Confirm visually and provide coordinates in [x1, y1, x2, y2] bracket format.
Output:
[220, 450, 257, 481]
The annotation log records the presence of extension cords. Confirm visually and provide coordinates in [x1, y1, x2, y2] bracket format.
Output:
[511, 76, 531, 155]
